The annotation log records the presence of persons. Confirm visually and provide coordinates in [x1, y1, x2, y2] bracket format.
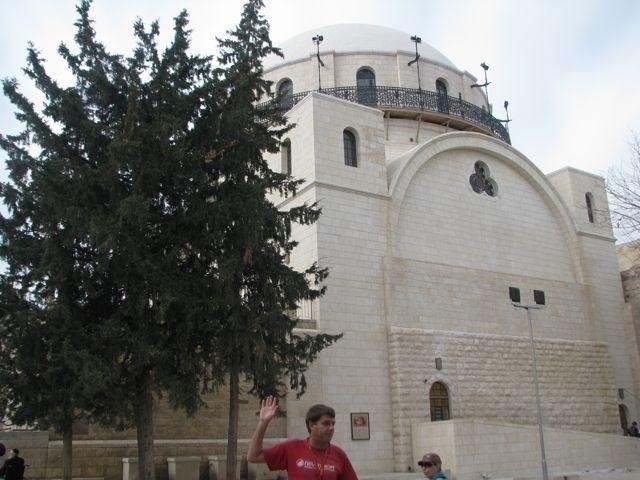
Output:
[628, 420, 640, 437]
[417, 452, 449, 480]
[247, 395, 358, 480]
[0, 447, 25, 480]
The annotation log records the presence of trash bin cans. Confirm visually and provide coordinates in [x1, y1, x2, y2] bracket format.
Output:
[208, 455, 241, 480]
[248, 462, 279, 480]
[122, 458, 140, 480]
[167, 456, 201, 480]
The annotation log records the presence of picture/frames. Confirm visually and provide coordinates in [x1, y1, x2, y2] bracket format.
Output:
[351, 413, 371, 441]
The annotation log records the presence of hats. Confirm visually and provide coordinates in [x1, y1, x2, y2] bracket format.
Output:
[418, 452, 442, 466]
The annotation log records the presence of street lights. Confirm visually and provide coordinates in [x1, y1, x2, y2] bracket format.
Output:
[509, 287, 549, 480]
